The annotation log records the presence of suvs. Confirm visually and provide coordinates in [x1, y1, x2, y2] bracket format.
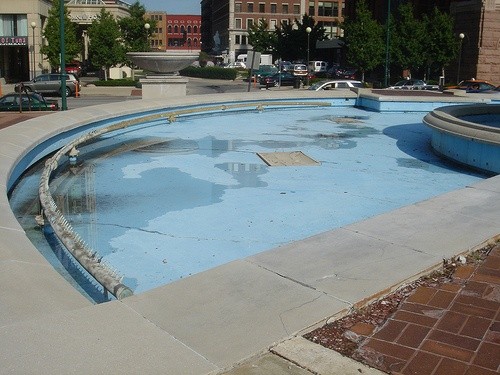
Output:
[14, 72, 82, 98]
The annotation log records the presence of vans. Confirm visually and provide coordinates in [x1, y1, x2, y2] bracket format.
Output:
[235, 53, 273, 64]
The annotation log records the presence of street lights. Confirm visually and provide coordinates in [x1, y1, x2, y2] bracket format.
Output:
[144, 23, 151, 44]
[30, 21, 36, 77]
[456, 33, 465, 86]
[306, 26, 312, 74]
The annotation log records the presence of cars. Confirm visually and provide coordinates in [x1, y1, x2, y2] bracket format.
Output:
[223, 61, 247, 70]
[454, 77, 500, 93]
[0, 91, 59, 111]
[242, 60, 358, 88]
[306, 79, 369, 91]
[386, 77, 428, 91]
[65, 60, 83, 77]
